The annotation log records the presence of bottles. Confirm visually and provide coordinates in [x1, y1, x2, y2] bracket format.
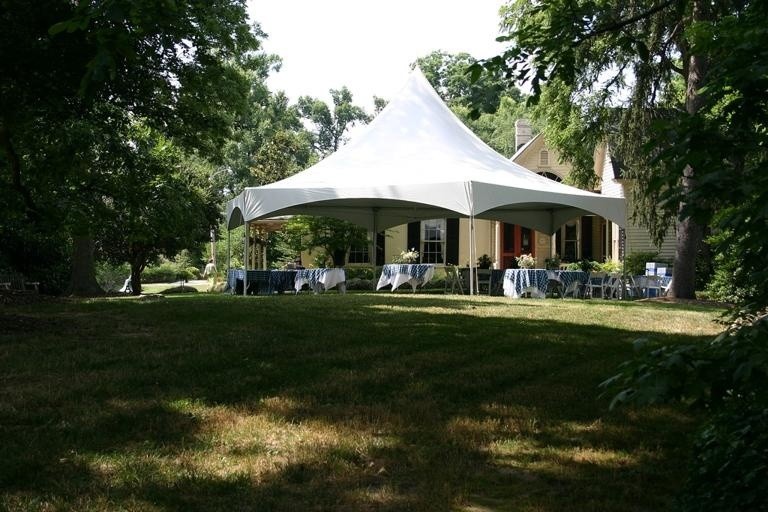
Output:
[645, 269, 649, 276]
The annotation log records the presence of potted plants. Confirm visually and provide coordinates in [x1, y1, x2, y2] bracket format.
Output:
[477, 253, 494, 269]
[313, 250, 330, 268]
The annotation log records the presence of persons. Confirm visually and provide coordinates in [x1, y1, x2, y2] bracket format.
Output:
[203, 259, 216, 280]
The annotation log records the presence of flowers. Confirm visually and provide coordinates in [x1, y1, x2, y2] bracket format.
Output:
[400, 247, 420, 264]
[515, 253, 539, 268]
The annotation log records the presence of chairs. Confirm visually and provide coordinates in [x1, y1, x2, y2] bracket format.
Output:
[587, 271, 664, 300]
[441, 264, 589, 302]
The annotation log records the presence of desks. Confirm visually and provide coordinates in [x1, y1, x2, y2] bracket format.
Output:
[227, 263, 348, 298]
[376, 264, 436, 292]
